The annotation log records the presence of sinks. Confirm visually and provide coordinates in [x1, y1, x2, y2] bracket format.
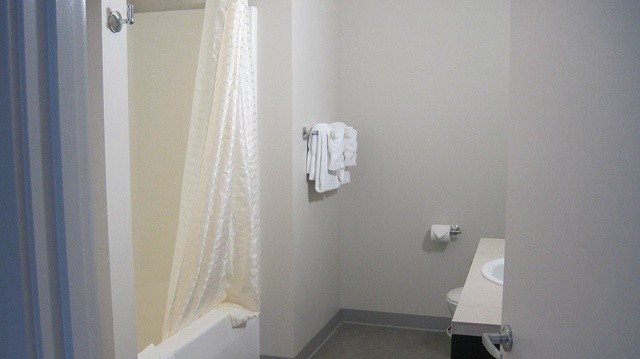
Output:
[482, 258, 504, 287]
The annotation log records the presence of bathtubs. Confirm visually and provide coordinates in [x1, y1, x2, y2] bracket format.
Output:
[138, 301, 259, 359]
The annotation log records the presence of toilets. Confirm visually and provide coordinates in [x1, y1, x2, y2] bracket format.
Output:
[446, 287, 464, 338]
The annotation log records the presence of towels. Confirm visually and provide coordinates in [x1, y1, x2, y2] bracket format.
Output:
[330, 121, 357, 185]
[307, 123, 339, 193]
